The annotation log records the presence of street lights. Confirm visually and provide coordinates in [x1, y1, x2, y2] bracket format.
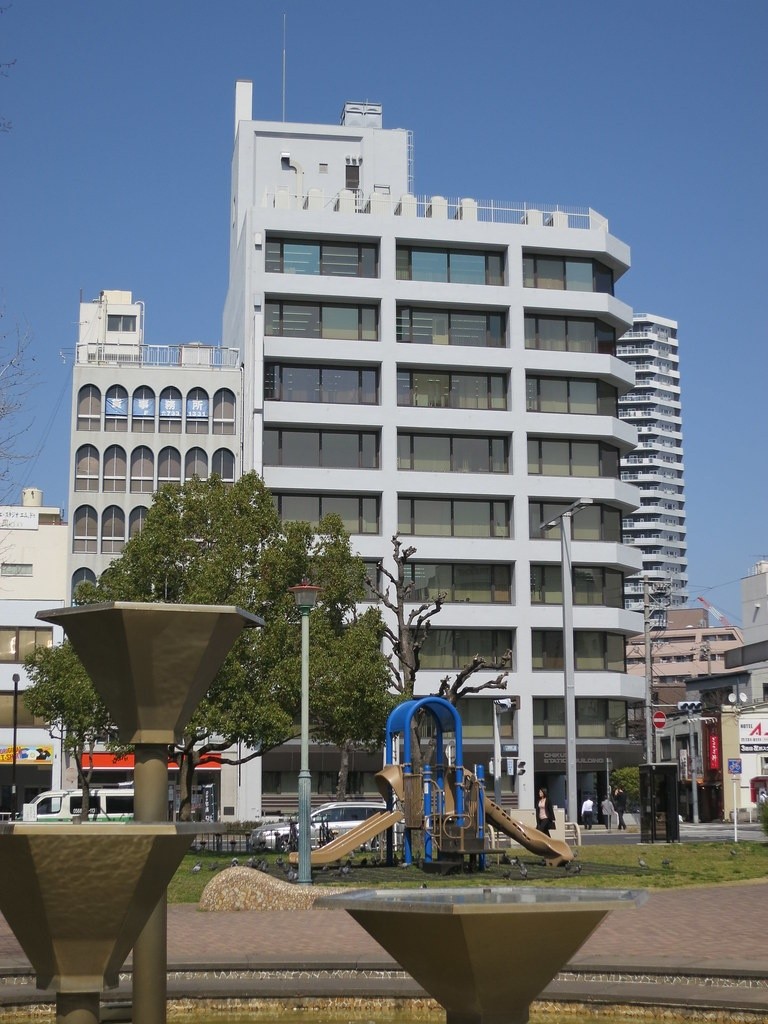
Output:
[10, 673, 23, 823]
[287, 573, 326, 886]
[538, 498, 595, 839]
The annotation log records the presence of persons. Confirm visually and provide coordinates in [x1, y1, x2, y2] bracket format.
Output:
[601, 795, 614, 829]
[581, 797, 594, 830]
[615, 787, 627, 830]
[759, 791, 768, 803]
[536, 787, 555, 837]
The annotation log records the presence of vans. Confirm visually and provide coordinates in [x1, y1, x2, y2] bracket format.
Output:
[7, 786, 136, 822]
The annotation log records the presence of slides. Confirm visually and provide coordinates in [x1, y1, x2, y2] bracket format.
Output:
[464, 792, 575, 868]
[289, 808, 404, 864]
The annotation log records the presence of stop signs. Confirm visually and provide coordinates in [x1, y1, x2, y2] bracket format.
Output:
[652, 710, 666, 729]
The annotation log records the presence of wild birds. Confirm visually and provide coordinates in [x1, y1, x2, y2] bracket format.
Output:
[662, 856, 670, 868]
[193, 850, 584, 888]
[638, 856, 649, 870]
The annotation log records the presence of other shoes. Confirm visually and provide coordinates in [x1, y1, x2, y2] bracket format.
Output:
[624, 825, 626, 829]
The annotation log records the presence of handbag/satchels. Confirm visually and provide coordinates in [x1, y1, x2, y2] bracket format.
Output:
[548, 822, 556, 830]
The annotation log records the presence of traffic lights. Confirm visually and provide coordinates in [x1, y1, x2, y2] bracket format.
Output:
[678, 701, 704, 711]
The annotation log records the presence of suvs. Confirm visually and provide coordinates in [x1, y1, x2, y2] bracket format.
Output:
[247, 802, 391, 854]
